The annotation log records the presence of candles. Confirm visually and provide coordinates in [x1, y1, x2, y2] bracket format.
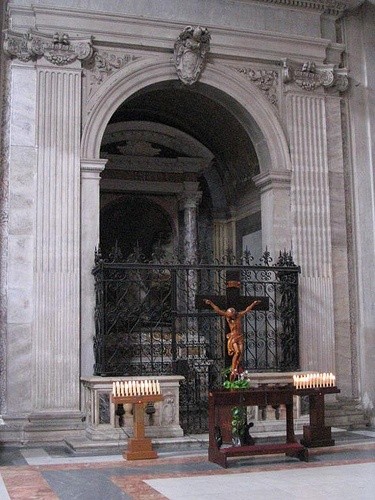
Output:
[112, 378, 161, 397]
[294, 371, 338, 390]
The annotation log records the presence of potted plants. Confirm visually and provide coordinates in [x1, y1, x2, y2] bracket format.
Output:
[223, 365, 257, 447]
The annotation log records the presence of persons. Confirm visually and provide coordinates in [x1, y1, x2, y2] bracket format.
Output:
[203, 298, 263, 385]
